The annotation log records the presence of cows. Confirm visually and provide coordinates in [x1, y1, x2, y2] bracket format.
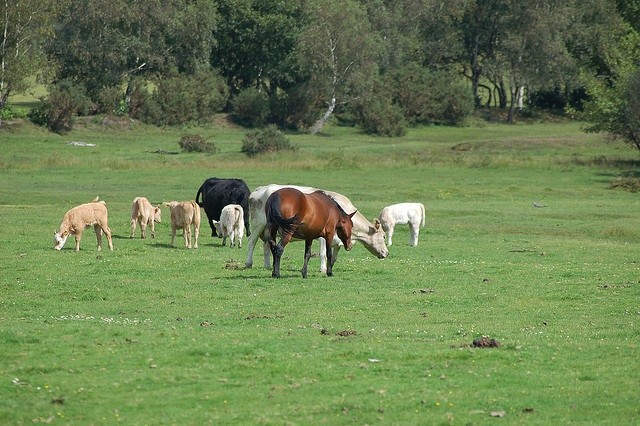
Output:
[377, 202, 426, 246]
[212, 204, 244, 248]
[52, 196, 113, 253]
[163, 200, 201, 249]
[245, 183, 389, 274]
[129, 196, 162, 239]
[196, 177, 251, 238]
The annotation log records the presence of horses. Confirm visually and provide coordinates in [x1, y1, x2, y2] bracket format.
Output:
[264, 187, 357, 279]
